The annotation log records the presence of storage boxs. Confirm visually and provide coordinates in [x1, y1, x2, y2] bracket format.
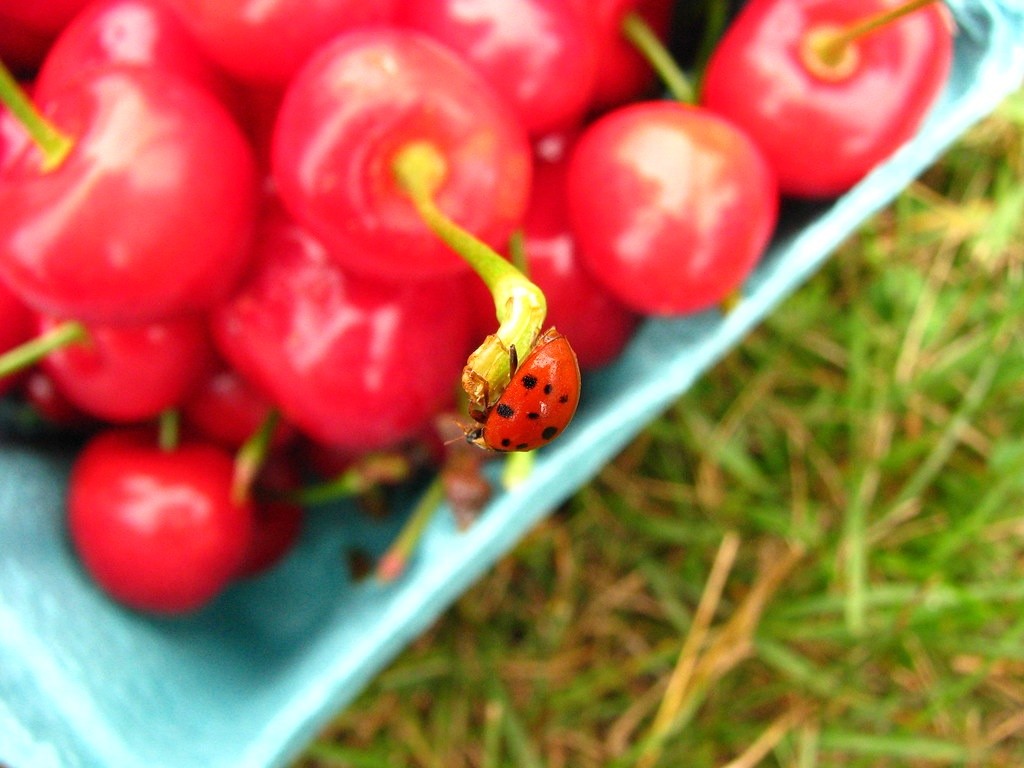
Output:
[0, 0, 1024, 768]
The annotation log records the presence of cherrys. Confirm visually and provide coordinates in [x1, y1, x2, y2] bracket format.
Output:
[0, 0, 956, 611]
